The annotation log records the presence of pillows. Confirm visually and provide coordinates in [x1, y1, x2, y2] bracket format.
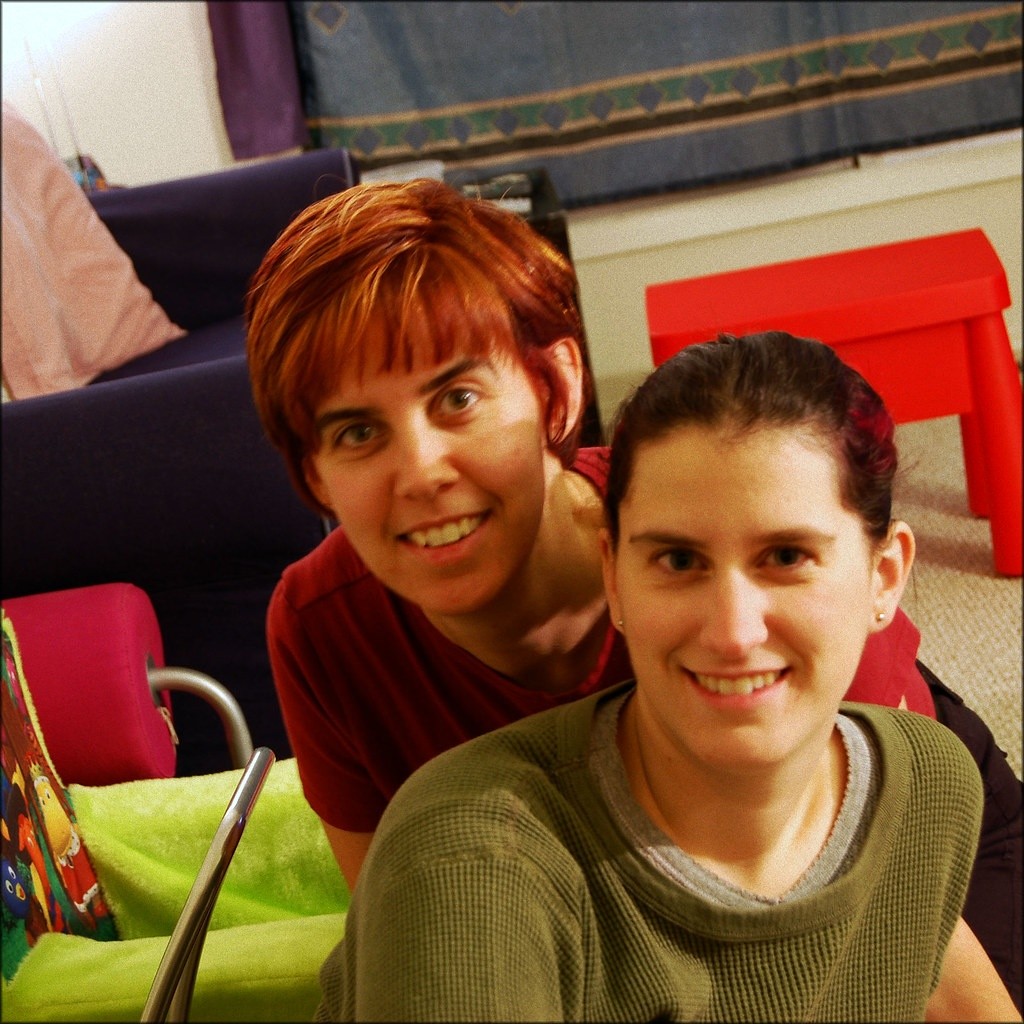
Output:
[0, 97, 189, 403]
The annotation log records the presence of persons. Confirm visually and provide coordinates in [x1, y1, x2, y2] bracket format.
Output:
[247, 179, 1024, 1024]
[1, 97, 189, 400]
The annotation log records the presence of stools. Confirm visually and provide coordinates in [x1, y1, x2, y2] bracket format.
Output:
[644, 228, 1024, 579]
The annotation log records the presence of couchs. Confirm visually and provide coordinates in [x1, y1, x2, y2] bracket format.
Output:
[0, 142, 607, 782]
